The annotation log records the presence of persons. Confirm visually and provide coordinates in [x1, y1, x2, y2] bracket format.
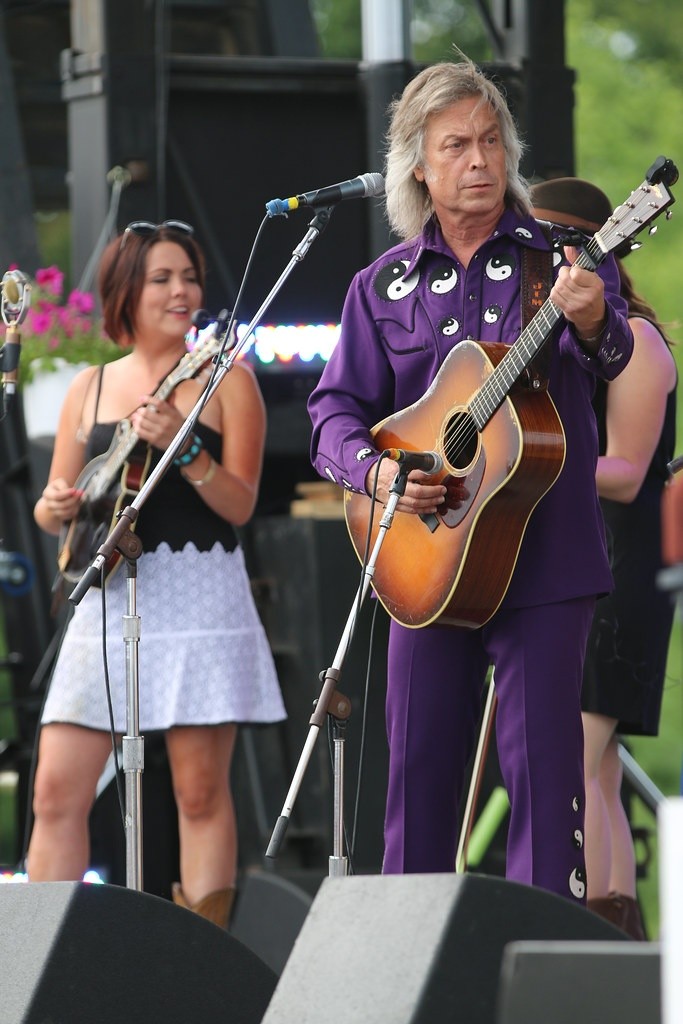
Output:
[307, 43, 679, 941]
[25, 221, 287, 933]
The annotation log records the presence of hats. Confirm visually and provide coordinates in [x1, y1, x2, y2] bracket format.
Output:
[529, 177, 633, 258]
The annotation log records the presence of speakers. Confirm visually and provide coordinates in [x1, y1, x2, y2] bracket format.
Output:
[0, 857, 667, 1024]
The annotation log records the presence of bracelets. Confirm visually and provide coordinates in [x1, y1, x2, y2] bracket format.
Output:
[180, 457, 216, 486]
[173, 436, 202, 467]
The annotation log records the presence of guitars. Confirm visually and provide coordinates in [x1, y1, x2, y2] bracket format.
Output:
[57, 307, 238, 587]
[340, 155, 680, 629]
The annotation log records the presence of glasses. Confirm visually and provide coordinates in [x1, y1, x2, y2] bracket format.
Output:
[119, 219, 193, 252]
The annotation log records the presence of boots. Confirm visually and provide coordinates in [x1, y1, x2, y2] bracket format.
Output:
[172, 883, 237, 930]
[588, 891, 644, 941]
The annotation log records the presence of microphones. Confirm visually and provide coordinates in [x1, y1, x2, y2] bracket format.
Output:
[0, 269, 31, 393]
[268, 173, 384, 215]
[384, 447, 444, 475]
